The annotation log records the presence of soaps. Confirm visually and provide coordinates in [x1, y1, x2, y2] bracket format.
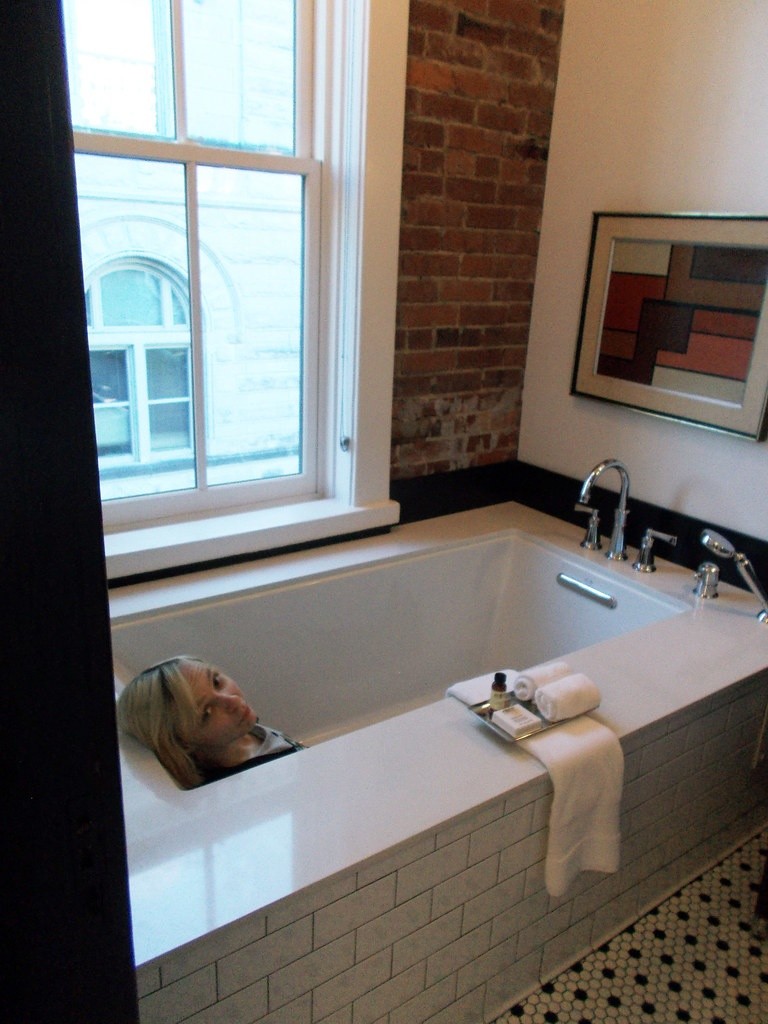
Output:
[490, 701, 546, 737]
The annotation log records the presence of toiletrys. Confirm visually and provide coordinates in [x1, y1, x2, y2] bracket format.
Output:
[486, 672, 509, 711]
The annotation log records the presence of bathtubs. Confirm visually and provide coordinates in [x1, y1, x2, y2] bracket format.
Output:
[75, 499, 766, 1022]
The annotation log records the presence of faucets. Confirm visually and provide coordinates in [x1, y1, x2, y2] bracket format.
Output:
[579, 456, 631, 563]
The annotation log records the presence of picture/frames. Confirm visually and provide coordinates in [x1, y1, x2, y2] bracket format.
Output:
[570, 208, 768, 443]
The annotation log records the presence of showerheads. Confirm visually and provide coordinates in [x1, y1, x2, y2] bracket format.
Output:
[699, 526, 768, 617]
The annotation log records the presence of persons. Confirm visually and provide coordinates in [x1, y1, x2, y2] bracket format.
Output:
[118, 654, 314, 788]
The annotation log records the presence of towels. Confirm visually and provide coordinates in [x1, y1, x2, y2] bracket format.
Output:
[441, 662, 625, 900]
